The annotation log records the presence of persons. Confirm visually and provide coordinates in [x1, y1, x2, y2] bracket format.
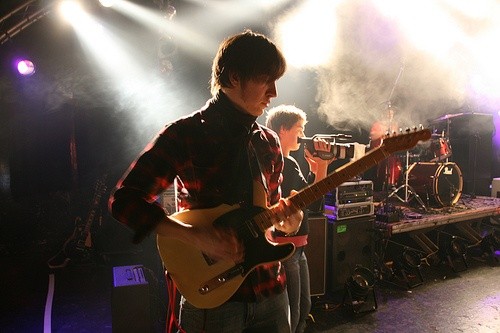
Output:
[108, 30, 304, 333]
[264, 105, 330, 333]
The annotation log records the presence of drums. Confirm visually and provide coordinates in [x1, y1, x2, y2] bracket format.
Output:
[418, 139, 453, 163]
[381, 156, 404, 186]
[405, 162, 463, 208]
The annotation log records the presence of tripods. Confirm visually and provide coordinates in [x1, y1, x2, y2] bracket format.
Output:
[380, 150, 429, 212]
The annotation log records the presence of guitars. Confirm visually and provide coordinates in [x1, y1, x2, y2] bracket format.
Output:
[62, 178, 108, 260]
[154, 123, 437, 309]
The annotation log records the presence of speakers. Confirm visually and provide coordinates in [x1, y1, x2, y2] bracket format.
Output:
[303, 215, 377, 297]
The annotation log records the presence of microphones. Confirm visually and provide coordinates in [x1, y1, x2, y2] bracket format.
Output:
[337, 134, 354, 140]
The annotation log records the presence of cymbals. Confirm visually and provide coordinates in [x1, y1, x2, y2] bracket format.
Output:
[435, 112, 464, 121]
